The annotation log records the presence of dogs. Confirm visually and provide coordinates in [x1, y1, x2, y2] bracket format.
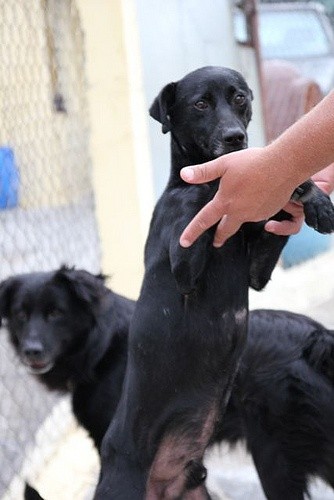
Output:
[0, 261, 334, 500]
[91, 67, 334, 500]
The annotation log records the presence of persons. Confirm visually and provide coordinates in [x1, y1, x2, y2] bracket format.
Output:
[178, 92, 334, 248]
